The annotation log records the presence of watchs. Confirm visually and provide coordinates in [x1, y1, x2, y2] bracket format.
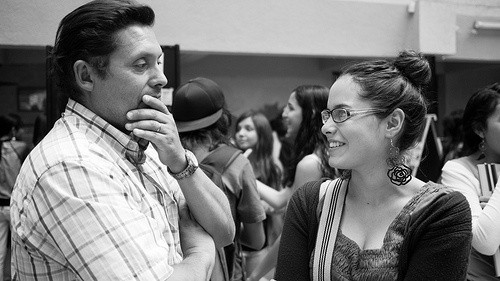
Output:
[167, 149, 199, 180]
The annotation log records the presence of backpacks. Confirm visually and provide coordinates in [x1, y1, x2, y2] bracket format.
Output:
[199, 145, 245, 281]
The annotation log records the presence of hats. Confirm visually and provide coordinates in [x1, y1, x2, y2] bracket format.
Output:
[174, 76, 226, 131]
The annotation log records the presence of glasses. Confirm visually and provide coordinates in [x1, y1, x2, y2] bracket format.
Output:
[321, 107, 386, 124]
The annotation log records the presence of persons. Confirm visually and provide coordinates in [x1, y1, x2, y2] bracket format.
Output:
[9, 1, 236, 281]
[270, 48, 474, 281]
[171, 78, 266, 281]
[441, 83, 500, 281]
[0, 113, 28, 281]
[232, 84, 347, 281]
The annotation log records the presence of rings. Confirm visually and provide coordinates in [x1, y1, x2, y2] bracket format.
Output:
[156, 123, 163, 132]
